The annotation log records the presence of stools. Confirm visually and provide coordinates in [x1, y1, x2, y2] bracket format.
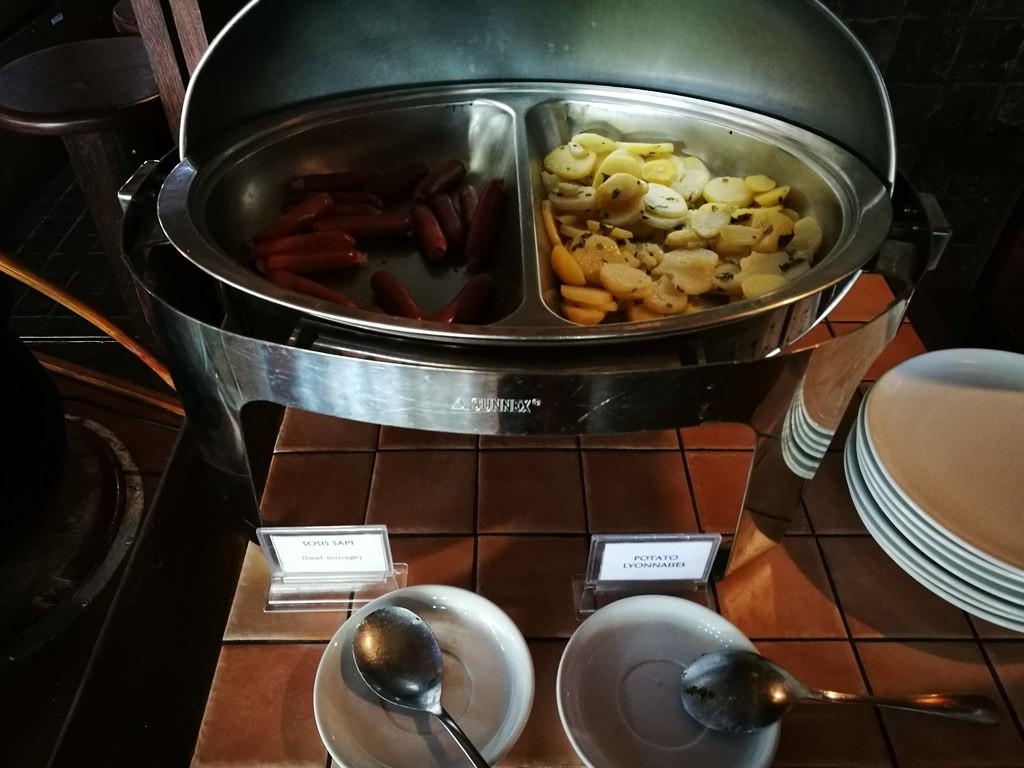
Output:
[0, 35, 189, 352]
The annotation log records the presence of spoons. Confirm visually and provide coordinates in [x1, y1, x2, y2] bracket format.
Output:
[680, 649, 1002, 734]
[353, 607, 492, 768]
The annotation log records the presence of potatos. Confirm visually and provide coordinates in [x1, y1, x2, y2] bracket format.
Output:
[542, 133, 822, 325]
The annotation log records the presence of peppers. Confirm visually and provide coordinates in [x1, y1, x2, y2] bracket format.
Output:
[244, 158, 507, 323]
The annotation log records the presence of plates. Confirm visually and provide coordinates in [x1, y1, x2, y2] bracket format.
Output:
[843, 348, 1024, 633]
[780, 299, 907, 479]
[314, 584, 535, 768]
[555, 595, 782, 768]
